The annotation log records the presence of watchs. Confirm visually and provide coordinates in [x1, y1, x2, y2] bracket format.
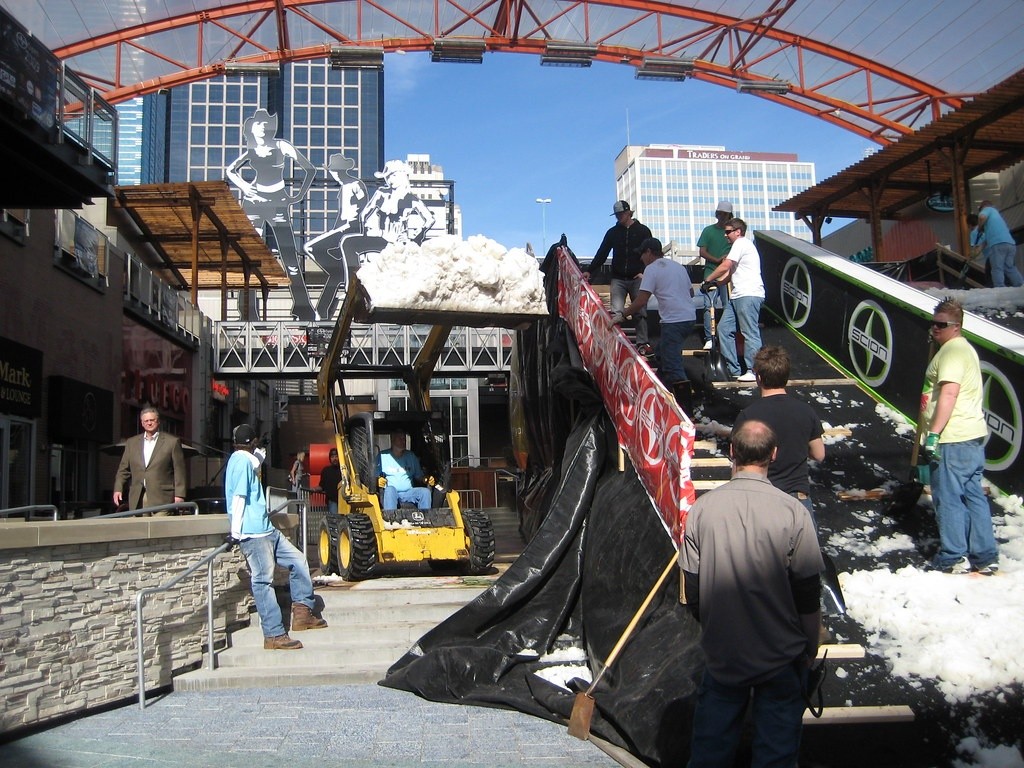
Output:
[704, 279, 709, 284]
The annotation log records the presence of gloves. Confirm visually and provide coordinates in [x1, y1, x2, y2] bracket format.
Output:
[425, 475, 434, 486]
[377, 477, 385, 488]
[699, 279, 711, 294]
[222, 535, 240, 552]
[921, 430, 941, 467]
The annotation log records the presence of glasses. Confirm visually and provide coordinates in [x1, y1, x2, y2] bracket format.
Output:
[930, 320, 961, 328]
[725, 228, 739, 235]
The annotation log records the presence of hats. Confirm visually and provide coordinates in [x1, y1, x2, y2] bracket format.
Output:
[716, 201, 733, 213]
[609, 200, 630, 216]
[233, 424, 256, 444]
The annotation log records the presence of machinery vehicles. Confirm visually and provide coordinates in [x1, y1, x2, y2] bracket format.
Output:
[316, 270, 551, 580]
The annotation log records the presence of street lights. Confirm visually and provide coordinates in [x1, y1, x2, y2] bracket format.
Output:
[536, 198, 552, 257]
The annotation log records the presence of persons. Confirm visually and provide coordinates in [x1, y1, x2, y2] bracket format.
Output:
[696, 202, 765, 382]
[607, 238, 696, 422]
[580, 200, 654, 357]
[320, 449, 342, 514]
[113, 407, 186, 518]
[290, 452, 306, 492]
[677, 419, 826, 768]
[730, 343, 825, 534]
[225, 423, 328, 650]
[966, 200, 1024, 287]
[375, 428, 435, 509]
[917, 296, 1000, 575]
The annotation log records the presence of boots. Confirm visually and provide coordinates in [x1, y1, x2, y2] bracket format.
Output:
[671, 380, 695, 421]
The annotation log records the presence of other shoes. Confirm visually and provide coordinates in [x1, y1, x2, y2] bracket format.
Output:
[971, 561, 999, 575]
[923, 555, 970, 574]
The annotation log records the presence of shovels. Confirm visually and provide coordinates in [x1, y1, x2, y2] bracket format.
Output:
[704, 284, 733, 382]
[568, 551, 680, 740]
[945, 228, 984, 288]
[886, 339, 938, 517]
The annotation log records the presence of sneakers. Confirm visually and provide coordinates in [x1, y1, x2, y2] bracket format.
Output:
[737, 370, 755, 381]
[635, 342, 654, 357]
[292, 603, 327, 630]
[264, 634, 302, 649]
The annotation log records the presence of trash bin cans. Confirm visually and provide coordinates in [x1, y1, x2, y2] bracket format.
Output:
[191, 498, 227, 513]
